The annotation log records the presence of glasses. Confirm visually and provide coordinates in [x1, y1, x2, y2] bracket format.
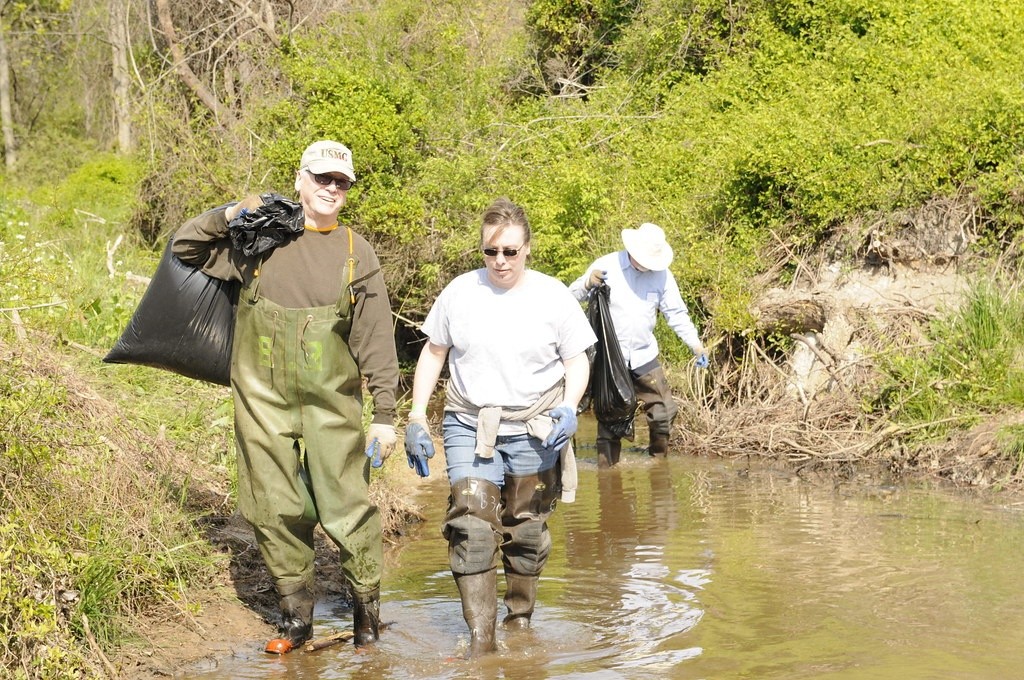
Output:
[480, 242, 526, 257]
[305, 169, 354, 191]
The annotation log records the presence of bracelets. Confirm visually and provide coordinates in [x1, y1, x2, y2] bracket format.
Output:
[410, 404, 426, 412]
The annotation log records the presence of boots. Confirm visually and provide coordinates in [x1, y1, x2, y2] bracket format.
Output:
[352, 587, 380, 654]
[265, 590, 313, 654]
[503, 573, 539, 632]
[448, 566, 497, 663]
[649, 429, 670, 458]
[595, 439, 621, 468]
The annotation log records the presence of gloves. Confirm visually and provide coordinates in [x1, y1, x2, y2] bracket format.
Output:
[225, 194, 264, 222]
[364, 424, 397, 468]
[586, 270, 608, 290]
[541, 402, 578, 452]
[405, 413, 435, 478]
[692, 346, 708, 369]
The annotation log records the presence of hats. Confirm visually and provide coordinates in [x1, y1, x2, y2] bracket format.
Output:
[621, 223, 673, 271]
[299, 141, 356, 181]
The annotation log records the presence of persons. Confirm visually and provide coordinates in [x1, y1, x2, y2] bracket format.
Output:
[405, 202, 600, 656]
[172, 141, 400, 654]
[569, 223, 708, 467]
